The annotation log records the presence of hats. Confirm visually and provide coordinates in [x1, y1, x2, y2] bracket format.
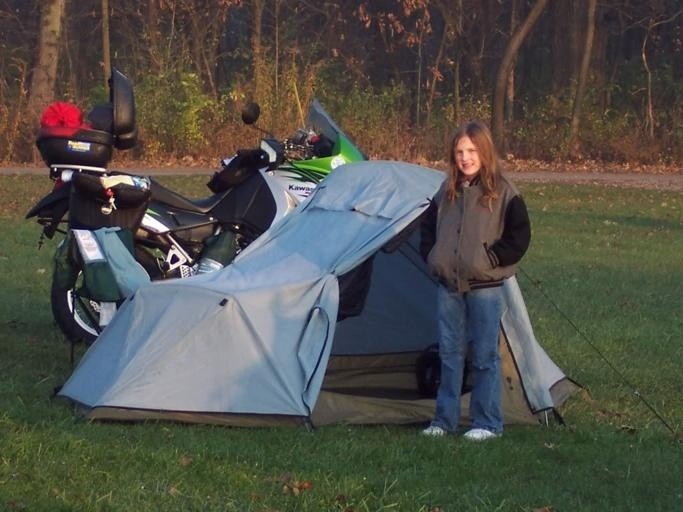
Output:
[40, 102, 82, 138]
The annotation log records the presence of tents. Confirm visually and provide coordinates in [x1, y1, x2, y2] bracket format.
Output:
[49, 157, 594, 434]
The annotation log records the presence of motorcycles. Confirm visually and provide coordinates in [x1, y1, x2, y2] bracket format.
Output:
[23, 66, 368, 348]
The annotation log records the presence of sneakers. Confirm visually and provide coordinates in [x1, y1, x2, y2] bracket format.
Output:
[422, 425, 503, 440]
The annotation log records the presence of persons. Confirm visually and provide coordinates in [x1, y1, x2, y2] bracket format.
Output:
[418, 122, 533, 444]
